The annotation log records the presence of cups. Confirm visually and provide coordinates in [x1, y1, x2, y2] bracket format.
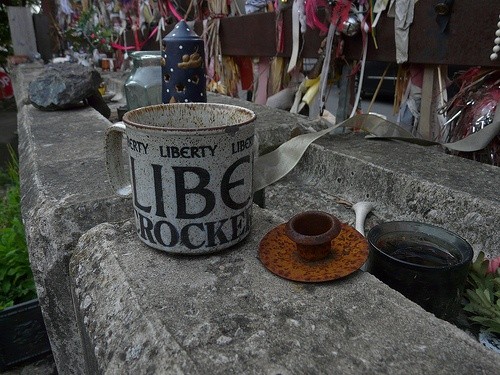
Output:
[368, 220, 473, 317]
[105, 102, 257, 253]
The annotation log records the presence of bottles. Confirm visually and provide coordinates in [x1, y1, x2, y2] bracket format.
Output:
[125, 50, 164, 110]
